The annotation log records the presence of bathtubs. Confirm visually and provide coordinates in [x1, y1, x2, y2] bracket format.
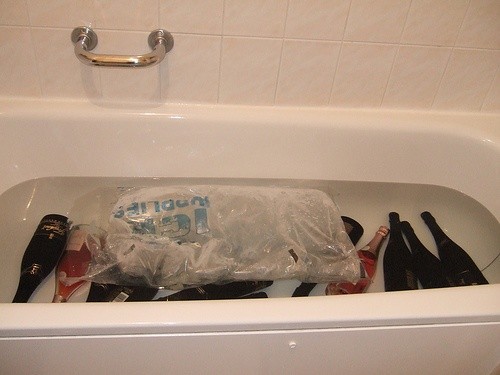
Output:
[0, 96, 500, 375]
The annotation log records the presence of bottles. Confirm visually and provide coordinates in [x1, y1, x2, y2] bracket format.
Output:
[155, 278, 273, 301]
[12, 214, 73, 303]
[383, 212, 417, 292]
[291, 216, 364, 297]
[401, 220, 455, 289]
[85, 255, 160, 302]
[52, 223, 107, 303]
[421, 211, 490, 286]
[323, 225, 390, 295]
